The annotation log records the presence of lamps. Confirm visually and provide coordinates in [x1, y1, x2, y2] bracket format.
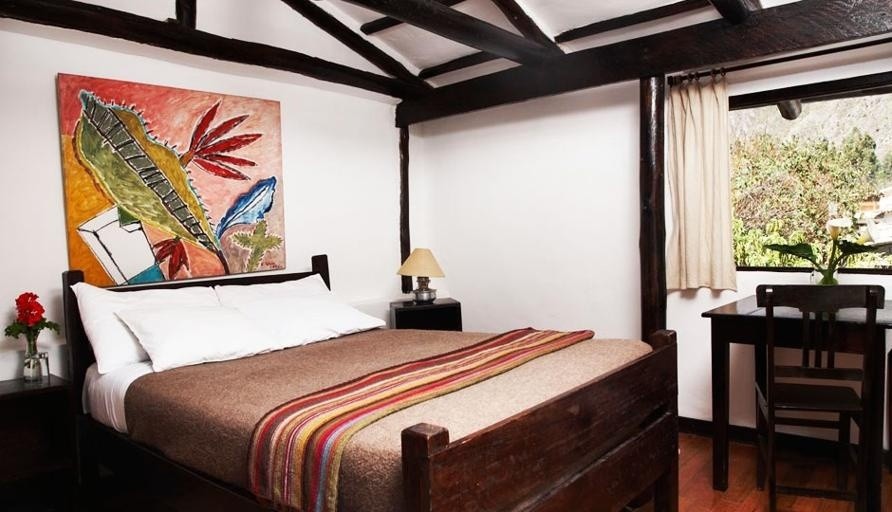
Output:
[397, 248, 444, 303]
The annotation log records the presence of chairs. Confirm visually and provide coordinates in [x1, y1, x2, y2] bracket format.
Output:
[753, 285, 884, 512]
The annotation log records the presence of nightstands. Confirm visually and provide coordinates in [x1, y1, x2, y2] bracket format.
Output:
[390, 297, 463, 331]
[0, 375, 85, 511]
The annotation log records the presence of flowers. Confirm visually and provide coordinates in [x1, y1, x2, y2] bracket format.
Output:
[5, 292, 61, 354]
[765, 219, 879, 285]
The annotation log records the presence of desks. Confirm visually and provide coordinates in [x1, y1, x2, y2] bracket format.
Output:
[702, 295, 892, 492]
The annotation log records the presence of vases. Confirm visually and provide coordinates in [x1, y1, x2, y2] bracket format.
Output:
[810, 263, 839, 284]
[23, 333, 42, 382]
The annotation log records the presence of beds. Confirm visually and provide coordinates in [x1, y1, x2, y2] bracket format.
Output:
[62, 254, 678, 512]
